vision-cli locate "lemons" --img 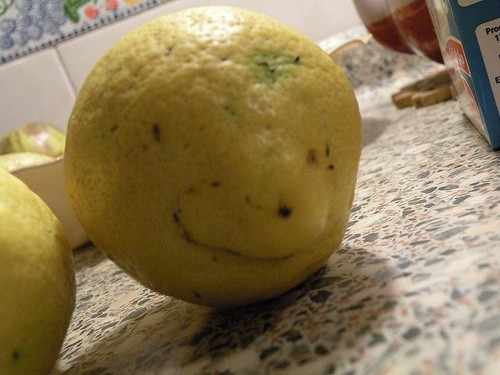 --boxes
[0,166,76,375]
[62,6,361,307]
